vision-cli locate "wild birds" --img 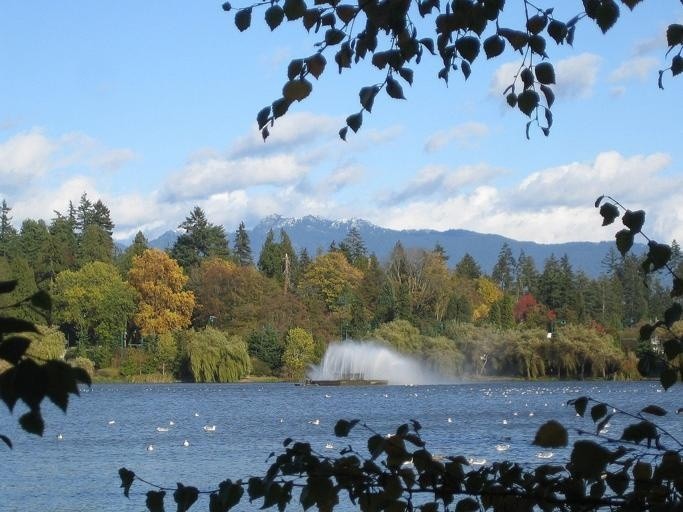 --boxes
[597,422,610,433]
[325,443,333,449]
[56,432,62,439]
[534,452,554,458]
[494,443,511,451]
[431,453,443,460]
[403,458,414,465]
[468,457,487,465]
[108,420,115,424]
[145,412,216,452]
[311,419,319,424]
